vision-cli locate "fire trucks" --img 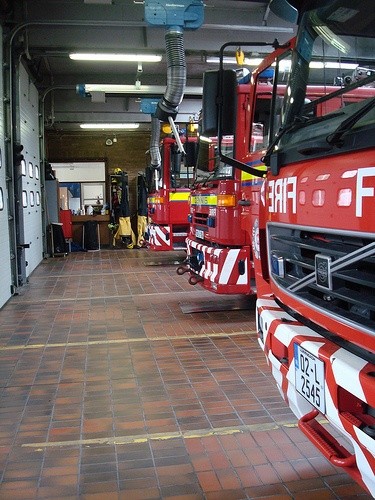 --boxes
[177,69,375,295]
[201,1,375,500]
[139,124,265,251]
